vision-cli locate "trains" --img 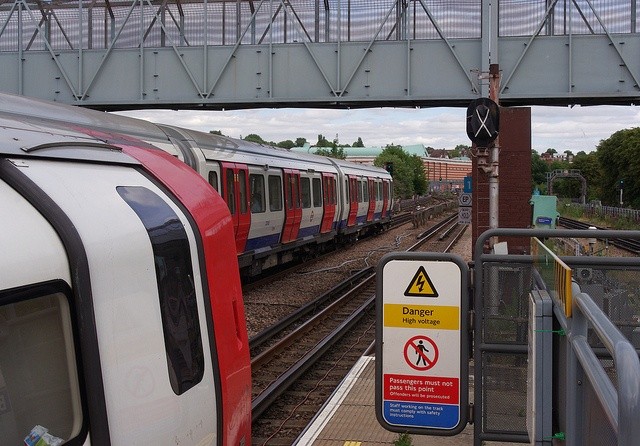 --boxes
[0,127,253,446]
[0,93,393,279]
